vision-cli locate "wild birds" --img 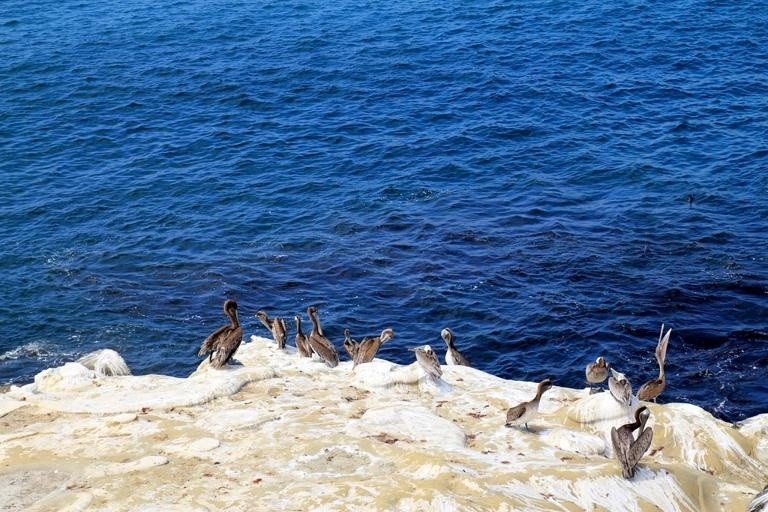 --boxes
[505,377,556,434]
[253,306,339,369]
[636,322,672,405]
[608,366,633,407]
[409,344,443,378]
[354,328,393,368]
[197,299,244,370]
[611,406,653,480]
[440,328,470,367]
[585,356,610,396]
[343,326,359,358]
